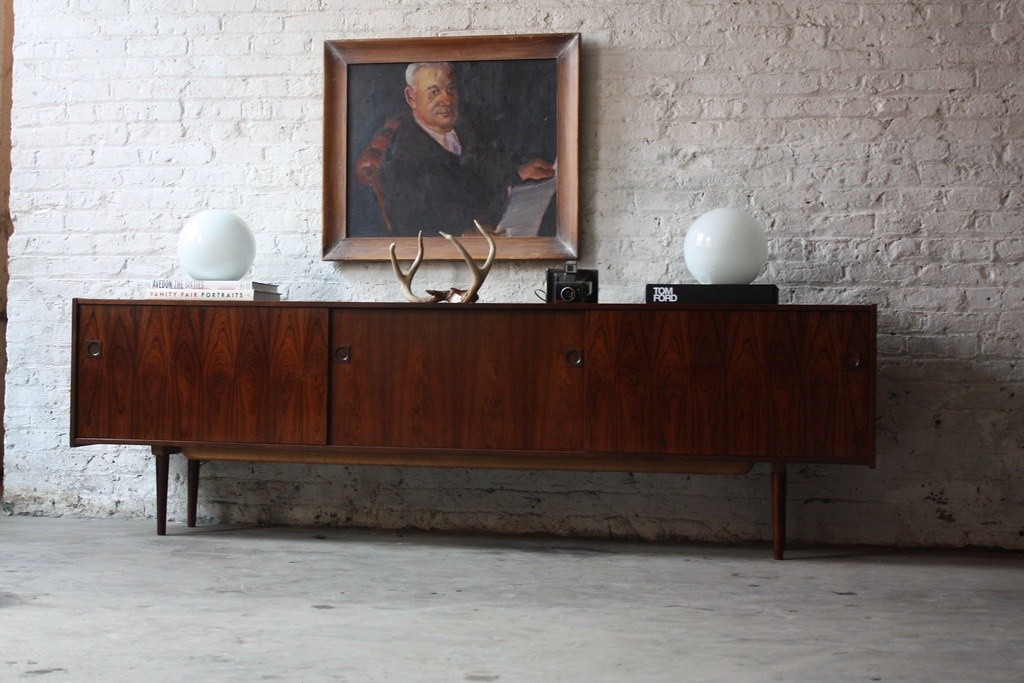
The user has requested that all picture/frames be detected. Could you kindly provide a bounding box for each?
[321,33,579,261]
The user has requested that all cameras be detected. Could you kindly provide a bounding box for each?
[545,261,599,302]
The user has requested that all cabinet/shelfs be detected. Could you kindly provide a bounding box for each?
[70,298,878,561]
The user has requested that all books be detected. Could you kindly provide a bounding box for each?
[148,279,283,300]
